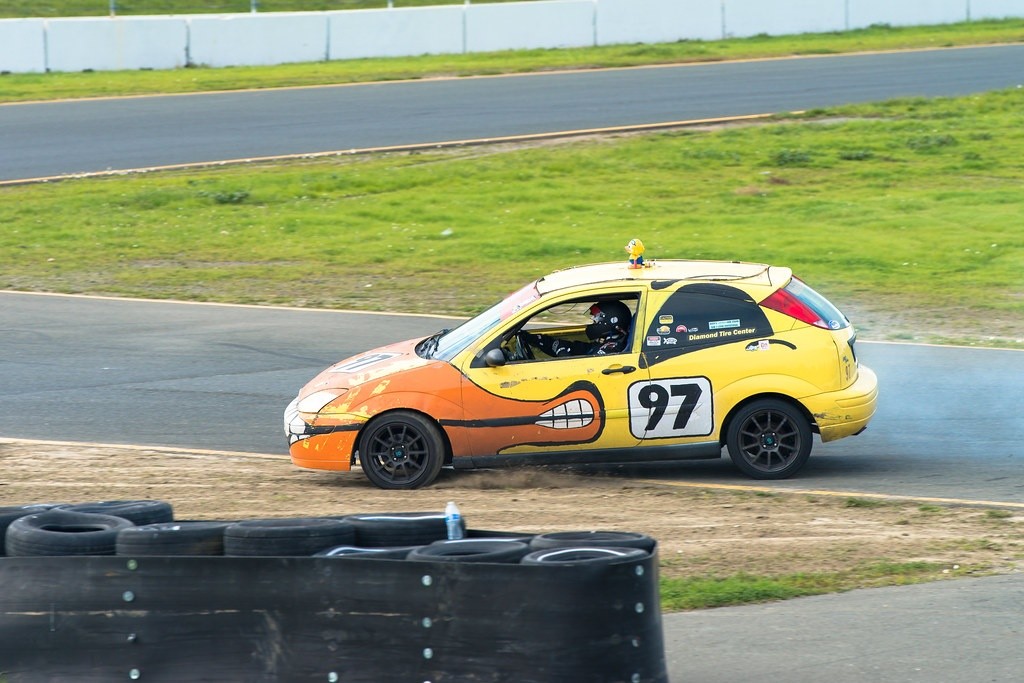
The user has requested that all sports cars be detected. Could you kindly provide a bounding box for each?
[282,237,880,491]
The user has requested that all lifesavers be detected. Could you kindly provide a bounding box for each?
[0,497,658,569]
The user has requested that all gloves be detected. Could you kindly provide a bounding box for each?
[515,330,537,346]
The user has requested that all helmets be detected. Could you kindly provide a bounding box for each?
[585,299,632,340]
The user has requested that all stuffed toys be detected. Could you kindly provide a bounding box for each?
[625,238,644,269]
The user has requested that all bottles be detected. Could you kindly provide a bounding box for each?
[444,501,463,540]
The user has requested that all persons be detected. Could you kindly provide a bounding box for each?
[519,301,633,357]
